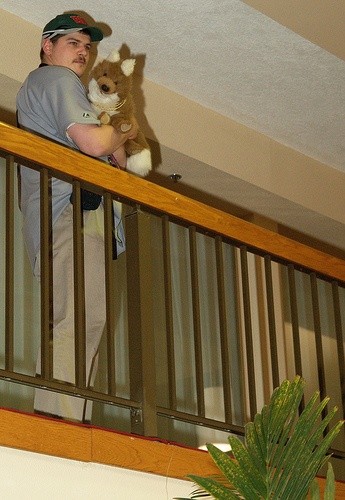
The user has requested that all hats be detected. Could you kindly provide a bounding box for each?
[42,14,103,41]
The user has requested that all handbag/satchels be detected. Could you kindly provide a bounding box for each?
[70,187,101,210]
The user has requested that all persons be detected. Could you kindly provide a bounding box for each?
[16,10,139,424]
